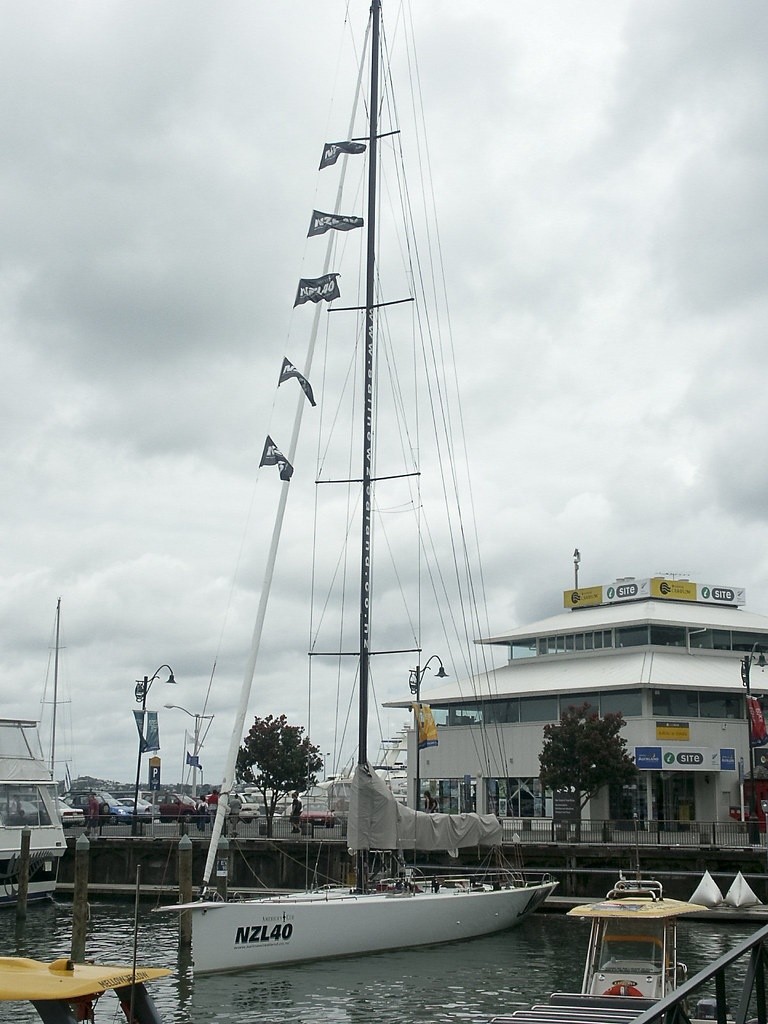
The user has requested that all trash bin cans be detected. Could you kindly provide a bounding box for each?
[556,827,567,841]
[342,820,347,836]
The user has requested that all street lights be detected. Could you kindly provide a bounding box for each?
[131,664,175,843]
[163,704,215,802]
[742,642,768,845]
[405,655,451,811]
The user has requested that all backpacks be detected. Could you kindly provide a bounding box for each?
[200,803,206,812]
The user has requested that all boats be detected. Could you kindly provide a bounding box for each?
[565,878,707,996]
[0,718,70,905]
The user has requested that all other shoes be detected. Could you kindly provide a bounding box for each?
[291,829,300,833]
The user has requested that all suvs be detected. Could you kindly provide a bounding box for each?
[57,790,134,824]
[230,792,260,823]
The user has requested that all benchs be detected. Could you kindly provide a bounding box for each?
[487,992,664,1024]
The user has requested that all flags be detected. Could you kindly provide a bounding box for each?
[292,273,341,309]
[186,750,198,766]
[186,732,204,748]
[278,357,317,407]
[319,141,366,169]
[306,210,364,238]
[259,435,294,481]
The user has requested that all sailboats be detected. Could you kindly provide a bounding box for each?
[152,0,564,978]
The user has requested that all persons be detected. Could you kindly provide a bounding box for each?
[290,792,303,833]
[198,791,242,835]
[424,791,437,813]
[86,794,97,837]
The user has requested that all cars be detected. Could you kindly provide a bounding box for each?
[137,792,209,822]
[117,797,153,817]
[300,798,334,828]
[24,800,85,827]
